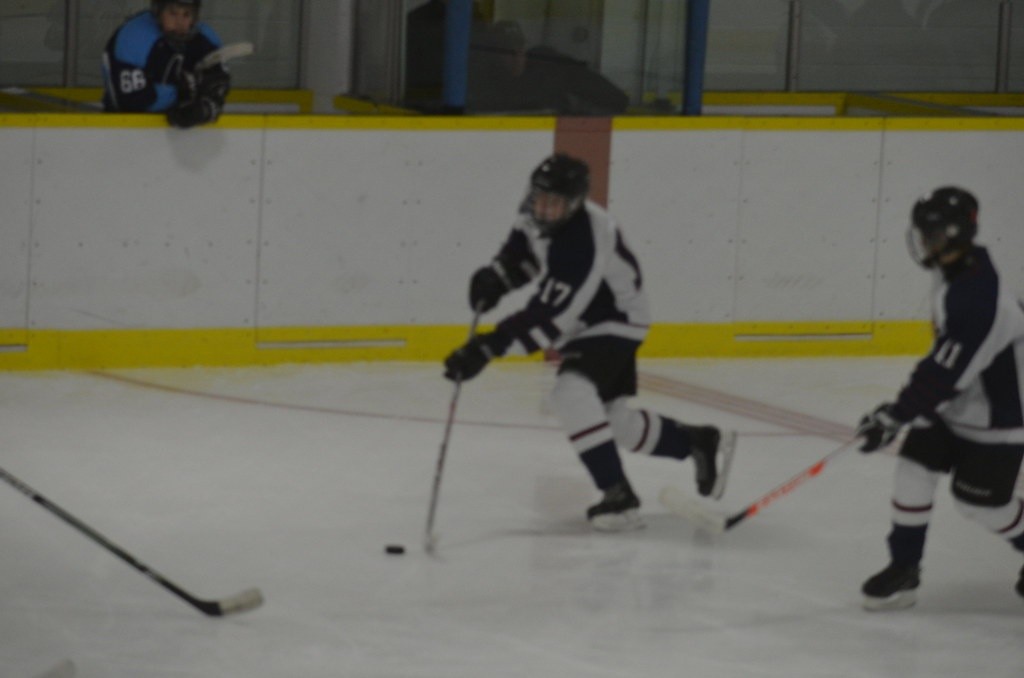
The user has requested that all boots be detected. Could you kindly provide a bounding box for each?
[628,410,738,500]
[995,498,1024,598]
[859,499,933,610]
[571,422,643,531]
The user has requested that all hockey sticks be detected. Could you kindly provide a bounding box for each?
[192,39,256,70]
[421,303,487,554]
[722,436,862,533]
[1,464,266,621]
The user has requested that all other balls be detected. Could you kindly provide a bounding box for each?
[385,545,404,554]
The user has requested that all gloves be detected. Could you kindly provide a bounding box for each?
[469,267,507,313]
[442,332,490,382]
[166,96,212,127]
[858,403,902,452]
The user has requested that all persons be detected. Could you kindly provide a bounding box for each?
[443,153,736,531]
[406,0,628,117]
[101,0,228,128]
[855,188,1024,610]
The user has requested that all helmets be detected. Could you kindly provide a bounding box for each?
[907,187,979,270]
[530,154,590,229]
[153,0,201,42]
